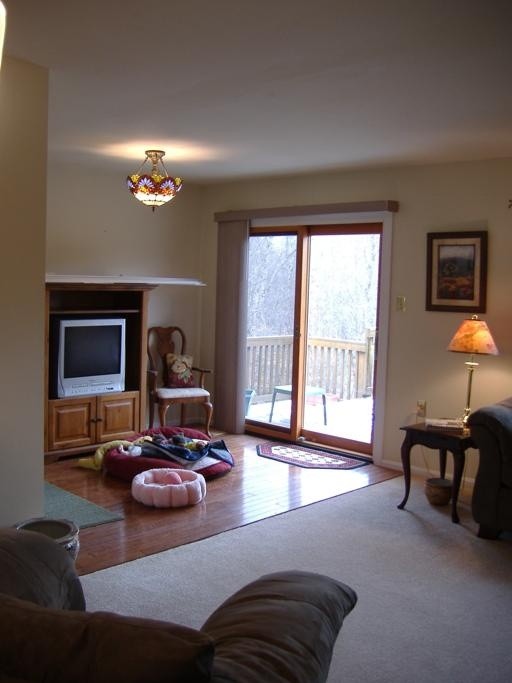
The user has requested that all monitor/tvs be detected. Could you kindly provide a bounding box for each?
[50,317,127,399]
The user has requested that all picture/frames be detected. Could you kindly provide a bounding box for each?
[422,230,487,315]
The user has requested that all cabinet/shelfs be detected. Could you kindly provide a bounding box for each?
[44,277,150,466]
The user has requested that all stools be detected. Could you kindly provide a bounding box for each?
[266,383,328,428]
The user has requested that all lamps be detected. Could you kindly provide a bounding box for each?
[441,316,501,429]
[123,145,186,214]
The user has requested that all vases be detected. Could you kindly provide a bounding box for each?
[424,477,453,510]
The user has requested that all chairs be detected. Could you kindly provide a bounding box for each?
[144,324,216,442]
[0,522,363,682]
[468,393,511,540]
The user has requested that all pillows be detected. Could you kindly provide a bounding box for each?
[163,351,197,388]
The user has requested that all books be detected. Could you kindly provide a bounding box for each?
[424,417,464,433]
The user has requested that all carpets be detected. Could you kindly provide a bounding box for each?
[78,470,512,683]
[256,439,372,471]
[43,476,126,533]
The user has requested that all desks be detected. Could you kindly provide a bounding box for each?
[396,421,477,528]
